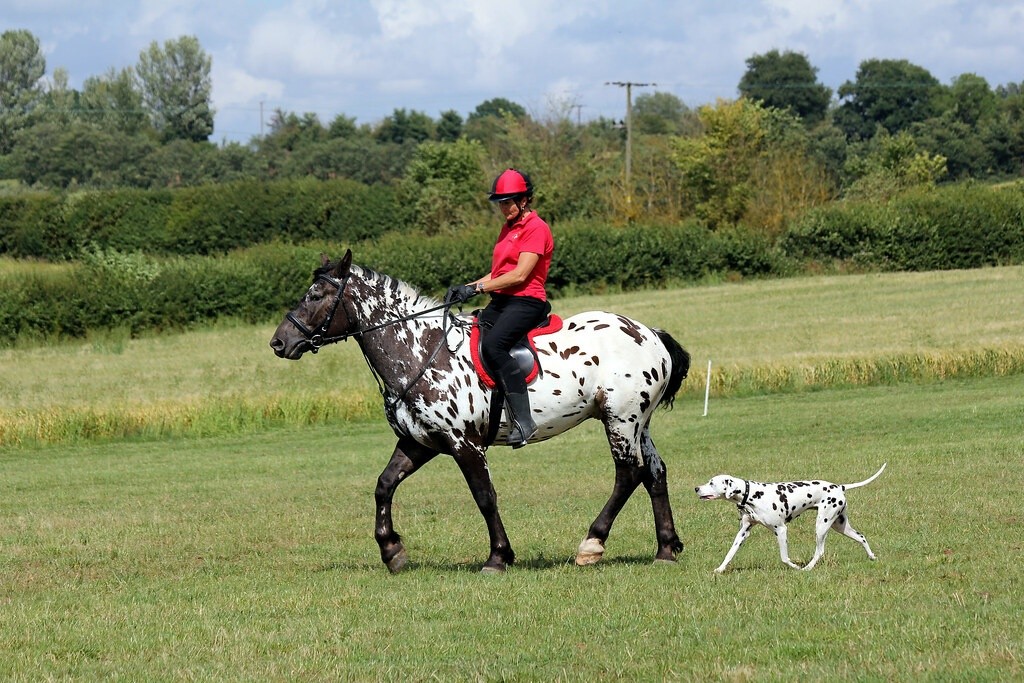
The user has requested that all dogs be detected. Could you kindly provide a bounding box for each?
[694,462,889,574]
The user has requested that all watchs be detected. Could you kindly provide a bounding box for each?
[478,282,485,294]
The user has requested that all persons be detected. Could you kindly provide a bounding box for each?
[442,166,554,447]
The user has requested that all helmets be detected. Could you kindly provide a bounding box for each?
[488,168,533,201]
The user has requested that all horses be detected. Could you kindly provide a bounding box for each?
[269,247,692,575]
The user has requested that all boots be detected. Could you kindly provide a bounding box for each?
[504,389,538,445]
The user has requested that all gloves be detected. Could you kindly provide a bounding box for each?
[445,283,478,303]
